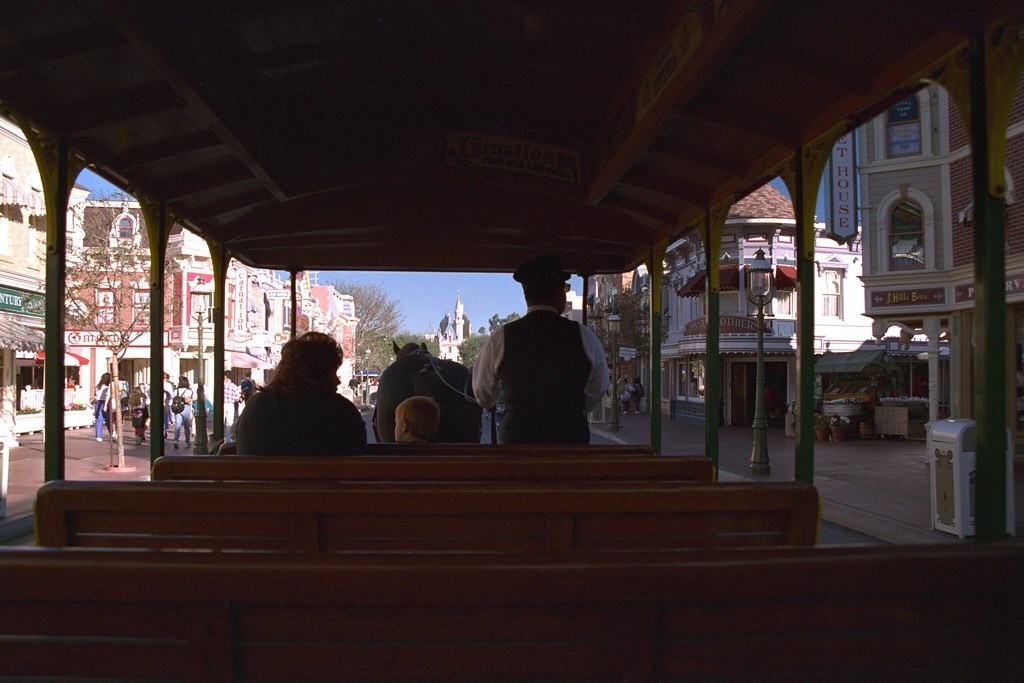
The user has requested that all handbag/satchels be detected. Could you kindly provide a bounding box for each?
[121,389,130,408]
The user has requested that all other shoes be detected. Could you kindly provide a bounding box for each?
[135,438,142,447]
[112,431,116,437]
[141,435,147,442]
[96,437,102,442]
[111,433,119,440]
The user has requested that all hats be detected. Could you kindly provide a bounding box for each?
[512,253,571,283]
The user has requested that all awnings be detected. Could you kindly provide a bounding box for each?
[225,351,274,370]
[0,317,70,353]
[677,266,796,298]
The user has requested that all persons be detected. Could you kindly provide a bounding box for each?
[394,396,441,443]
[224,370,239,441]
[230,331,367,456]
[172,376,197,449]
[349,376,358,397]
[103,371,130,437]
[192,398,213,412]
[191,380,198,437]
[94,374,117,441]
[471,258,610,445]
[163,372,175,429]
[127,383,150,445]
[765,383,776,419]
[234,370,257,419]
[621,378,644,414]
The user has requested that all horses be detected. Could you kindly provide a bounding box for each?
[373,341,483,443]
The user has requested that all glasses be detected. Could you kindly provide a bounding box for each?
[556,282,572,293]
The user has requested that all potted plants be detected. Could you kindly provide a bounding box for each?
[814,417,830,442]
[859,411,876,438]
[830,415,850,442]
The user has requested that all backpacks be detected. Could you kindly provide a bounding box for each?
[172,388,186,413]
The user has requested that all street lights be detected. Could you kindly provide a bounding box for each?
[608,314,622,433]
[745,246,774,479]
[364,349,372,410]
[187,273,214,456]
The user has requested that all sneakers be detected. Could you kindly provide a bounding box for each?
[184,442,191,448]
[635,411,641,414]
[623,410,628,415]
[173,439,179,449]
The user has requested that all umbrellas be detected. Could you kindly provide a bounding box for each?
[15,349,89,366]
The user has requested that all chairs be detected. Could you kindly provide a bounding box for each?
[0,442,1024,683]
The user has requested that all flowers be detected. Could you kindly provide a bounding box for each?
[17,403,87,415]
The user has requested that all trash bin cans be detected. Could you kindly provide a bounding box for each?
[341,389,353,403]
[929,418,1015,540]
[0,418,10,518]
